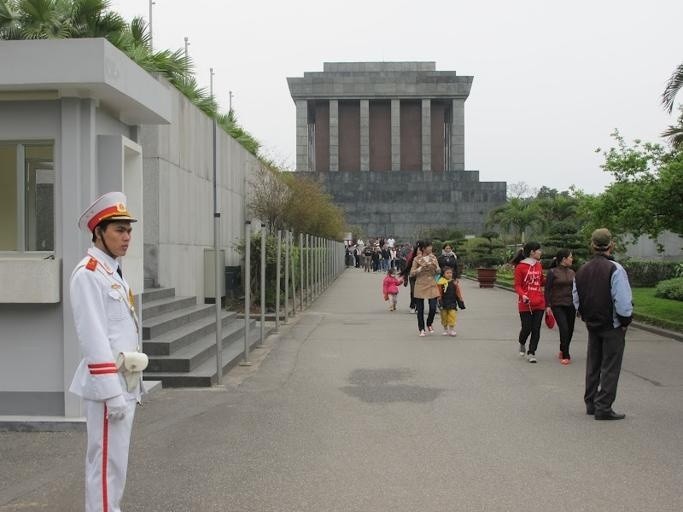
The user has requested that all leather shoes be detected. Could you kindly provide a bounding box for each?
[595,410,625,421]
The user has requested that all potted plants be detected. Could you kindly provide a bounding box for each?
[468,229,509,289]
[431,227,467,278]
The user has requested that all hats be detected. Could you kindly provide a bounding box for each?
[591,228,612,249]
[78,191,138,234]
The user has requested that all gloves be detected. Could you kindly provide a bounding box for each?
[105,395,127,421]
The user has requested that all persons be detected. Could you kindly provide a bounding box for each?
[570,227,633,420]
[67,190,150,511]
[343,235,467,339]
[542,249,581,367]
[508,240,547,364]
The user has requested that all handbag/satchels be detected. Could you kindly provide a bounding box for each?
[545,311,554,328]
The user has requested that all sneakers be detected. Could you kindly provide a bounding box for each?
[527,353,536,362]
[419,330,424,336]
[449,330,456,336]
[519,343,525,356]
[442,329,448,335]
[427,326,432,332]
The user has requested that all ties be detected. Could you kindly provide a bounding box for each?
[117,265,122,279]
